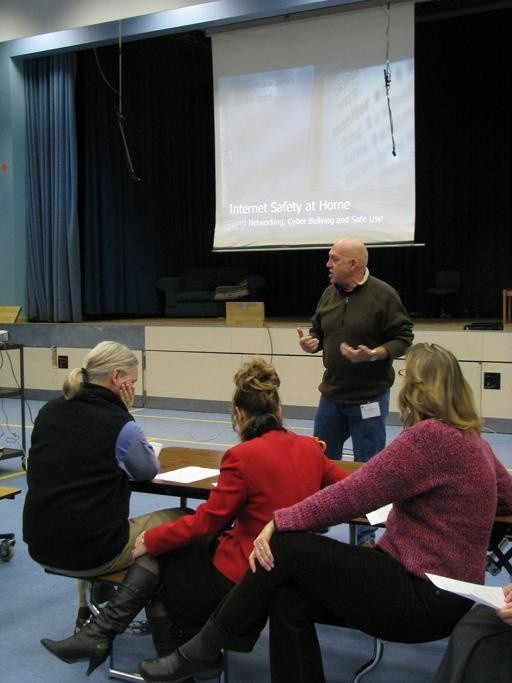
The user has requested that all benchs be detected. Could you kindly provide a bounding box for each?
[44,563,383,681]
[2,486,23,564]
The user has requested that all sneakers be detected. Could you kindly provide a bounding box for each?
[74,616,89,637]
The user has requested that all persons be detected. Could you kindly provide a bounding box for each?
[19,338,196,634]
[296,236,414,550]
[138,339,511,683]
[430,572,512,683]
[40,353,356,681]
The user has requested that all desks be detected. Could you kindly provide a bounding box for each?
[129,446,510,577]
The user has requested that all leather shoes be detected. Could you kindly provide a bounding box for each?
[138,649,225,682]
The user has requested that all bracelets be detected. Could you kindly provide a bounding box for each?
[370,349,378,362]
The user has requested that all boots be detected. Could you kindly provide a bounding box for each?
[39,560,160,677]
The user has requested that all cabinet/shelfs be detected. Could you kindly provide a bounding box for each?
[1,330,28,472]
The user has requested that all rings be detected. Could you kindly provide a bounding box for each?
[257,546,267,551]
